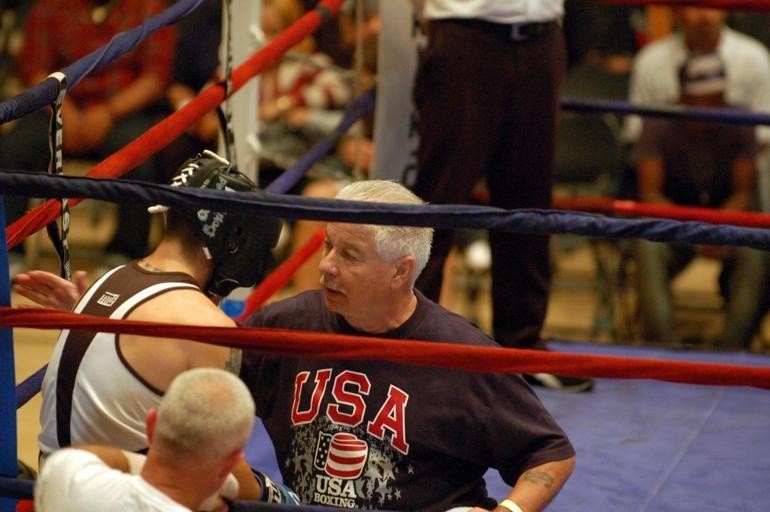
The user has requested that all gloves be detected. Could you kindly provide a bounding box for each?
[249,467,301,506]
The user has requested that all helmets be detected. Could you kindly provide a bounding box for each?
[149,148,285,297]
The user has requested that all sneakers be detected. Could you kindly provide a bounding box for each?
[523,371,594,394]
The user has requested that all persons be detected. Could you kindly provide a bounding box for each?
[1,0,770,512]
